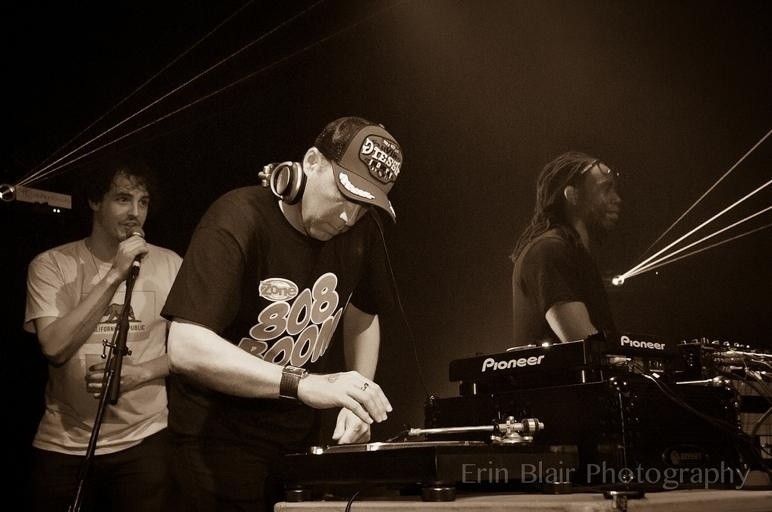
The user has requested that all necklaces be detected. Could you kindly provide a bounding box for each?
[87,244,102,281]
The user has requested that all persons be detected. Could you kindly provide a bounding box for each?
[160,117,403,512]
[21,164,184,512]
[510,151,625,357]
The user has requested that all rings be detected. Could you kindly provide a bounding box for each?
[361,383,368,392]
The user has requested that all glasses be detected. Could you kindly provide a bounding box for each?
[580,158,624,180]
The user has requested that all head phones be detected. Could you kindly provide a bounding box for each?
[270,158,308,204]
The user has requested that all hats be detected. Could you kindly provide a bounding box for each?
[314,116,403,224]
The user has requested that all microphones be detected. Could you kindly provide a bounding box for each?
[126,226,145,273]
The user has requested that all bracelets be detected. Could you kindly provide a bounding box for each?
[278,365,308,405]
